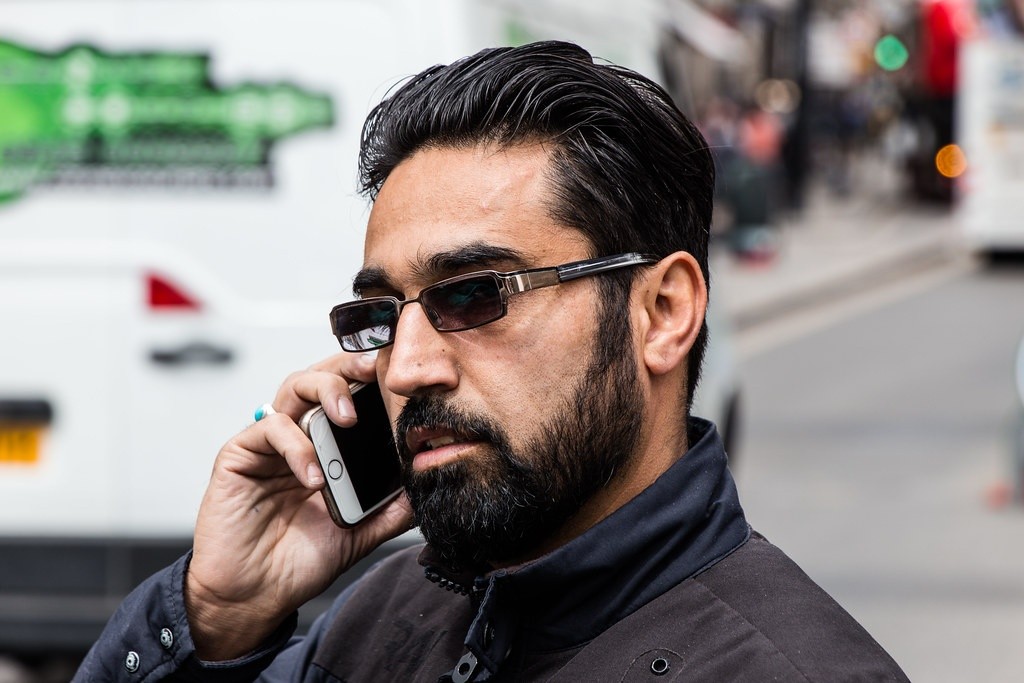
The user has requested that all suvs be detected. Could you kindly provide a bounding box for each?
[0,160,748,657]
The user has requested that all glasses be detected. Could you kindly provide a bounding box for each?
[328,249,666,354]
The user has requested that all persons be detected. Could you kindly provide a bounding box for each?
[69,41,914,683]
[702,83,952,264]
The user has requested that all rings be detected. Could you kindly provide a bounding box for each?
[253,402,277,422]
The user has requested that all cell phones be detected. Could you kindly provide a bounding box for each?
[299,380,406,530]
